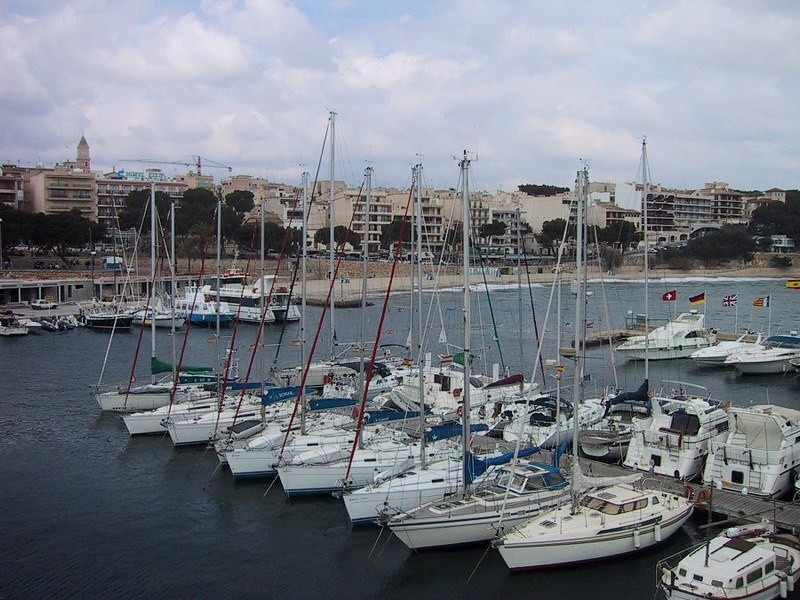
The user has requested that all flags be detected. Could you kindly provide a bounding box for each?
[721,294,737,306]
[402,358,414,366]
[785,280,800,290]
[662,290,676,302]
[688,292,705,306]
[441,355,453,364]
[555,364,564,372]
[752,295,769,307]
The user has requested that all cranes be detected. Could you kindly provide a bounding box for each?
[118,156,231,176]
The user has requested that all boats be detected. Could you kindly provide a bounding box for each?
[171,280,232,324]
[690,331,763,365]
[722,331,800,375]
[656,480,800,600]
[615,310,716,361]
[705,402,800,501]
[303,354,616,451]
[620,379,728,484]
[0,311,84,335]
[211,252,300,324]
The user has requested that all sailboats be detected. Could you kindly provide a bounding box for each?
[270,164,467,498]
[87,188,134,328]
[579,137,660,462]
[339,161,523,522]
[377,150,611,549]
[94,107,371,478]
[126,206,186,330]
[489,165,696,570]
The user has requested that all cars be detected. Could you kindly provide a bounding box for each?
[31,300,58,310]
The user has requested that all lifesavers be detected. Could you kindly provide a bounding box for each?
[454,388,460,397]
[697,490,711,510]
[457,406,463,417]
[685,486,694,499]
